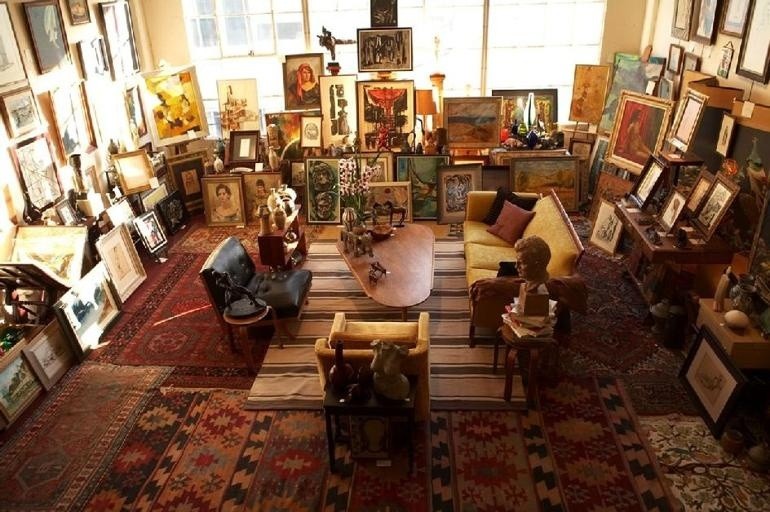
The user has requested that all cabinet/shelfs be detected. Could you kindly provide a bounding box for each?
[615,200,734,326]
[692,295,769,370]
[256,204,308,269]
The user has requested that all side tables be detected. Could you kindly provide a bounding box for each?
[493,327,553,402]
[222,302,283,374]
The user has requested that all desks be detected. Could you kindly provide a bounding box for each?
[321,377,417,473]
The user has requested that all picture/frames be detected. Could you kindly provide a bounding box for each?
[65,2,93,26]
[136,211,168,255]
[289,159,305,188]
[243,172,284,227]
[48,83,94,161]
[74,165,103,194]
[370,0,399,28]
[304,155,342,226]
[201,176,245,227]
[51,260,124,366]
[98,2,139,81]
[338,150,394,224]
[718,1,752,38]
[568,64,610,126]
[693,0,722,46]
[491,89,559,147]
[0,338,43,423]
[665,87,710,156]
[139,65,210,149]
[75,36,108,82]
[142,183,170,234]
[603,88,674,178]
[21,318,77,391]
[666,44,681,75]
[57,201,78,227]
[627,155,669,214]
[681,169,716,219]
[319,74,358,154]
[168,156,211,215]
[396,155,449,220]
[441,96,504,149]
[681,52,701,82]
[0,86,43,141]
[677,324,750,443]
[283,53,326,112]
[735,1,770,85]
[106,197,138,237]
[110,149,156,197]
[496,151,568,167]
[229,130,260,164]
[689,170,741,242]
[585,169,634,226]
[362,181,414,222]
[438,162,485,226]
[95,222,147,305]
[509,155,581,214]
[125,83,152,149]
[23,1,73,75]
[715,110,737,160]
[671,0,695,42]
[300,115,324,149]
[355,79,415,154]
[657,186,687,238]
[217,79,260,143]
[7,132,65,214]
[587,198,623,257]
[356,27,414,72]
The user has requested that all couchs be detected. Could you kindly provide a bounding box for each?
[313,310,432,417]
[462,191,585,348]
[199,237,312,350]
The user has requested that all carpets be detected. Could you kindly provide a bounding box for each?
[246,240,529,410]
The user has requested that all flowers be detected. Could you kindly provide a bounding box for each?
[340,153,383,207]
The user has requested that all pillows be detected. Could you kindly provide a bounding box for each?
[485,199,535,247]
[479,186,536,226]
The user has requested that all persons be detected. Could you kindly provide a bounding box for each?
[256,179,270,208]
[514,235,551,316]
[213,184,240,221]
[628,110,651,160]
[288,63,319,107]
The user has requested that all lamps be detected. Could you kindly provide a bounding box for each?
[416,89,434,152]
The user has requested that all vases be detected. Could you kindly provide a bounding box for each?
[342,206,357,226]
